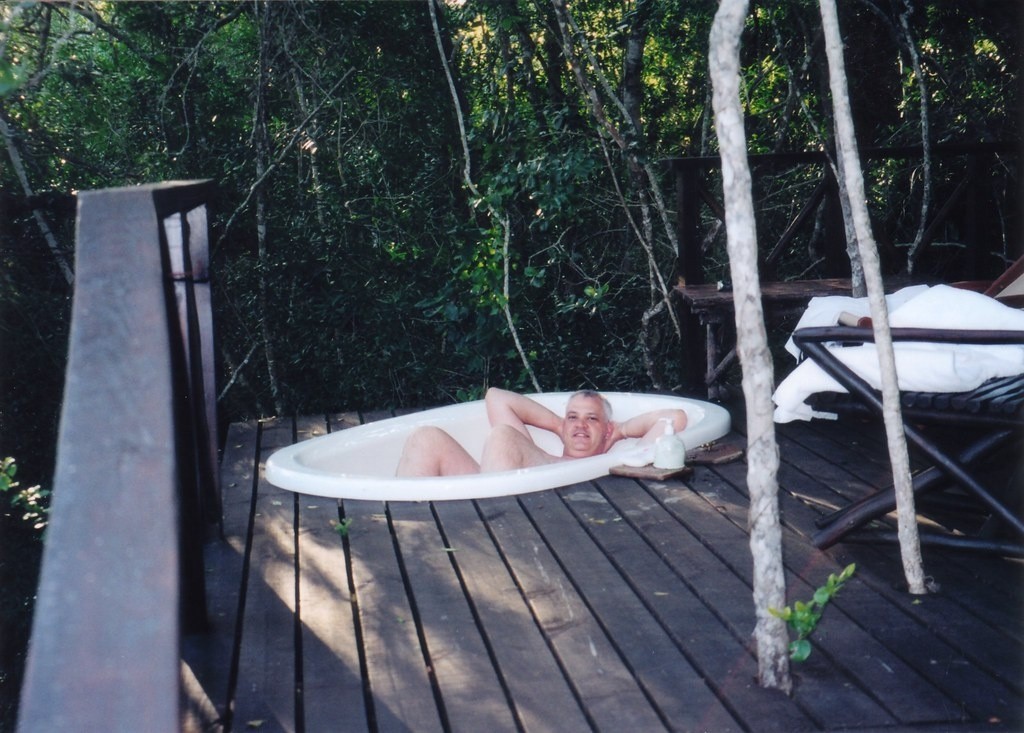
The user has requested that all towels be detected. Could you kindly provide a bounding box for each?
[769,283,1023,426]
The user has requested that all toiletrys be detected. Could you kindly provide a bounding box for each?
[653,418,686,469]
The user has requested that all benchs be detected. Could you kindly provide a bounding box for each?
[660,143,1006,407]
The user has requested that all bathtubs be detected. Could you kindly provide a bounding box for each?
[263,388,730,500]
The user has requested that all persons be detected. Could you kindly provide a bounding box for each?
[396,388,688,478]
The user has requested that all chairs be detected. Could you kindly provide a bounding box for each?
[791,291,1024,557]
